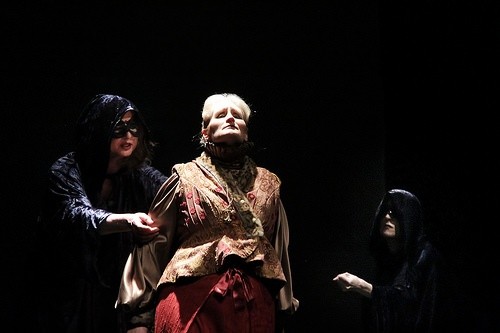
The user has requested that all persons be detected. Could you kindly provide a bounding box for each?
[333,189,459,333]
[47,94,169,333]
[114,93,299,333]
